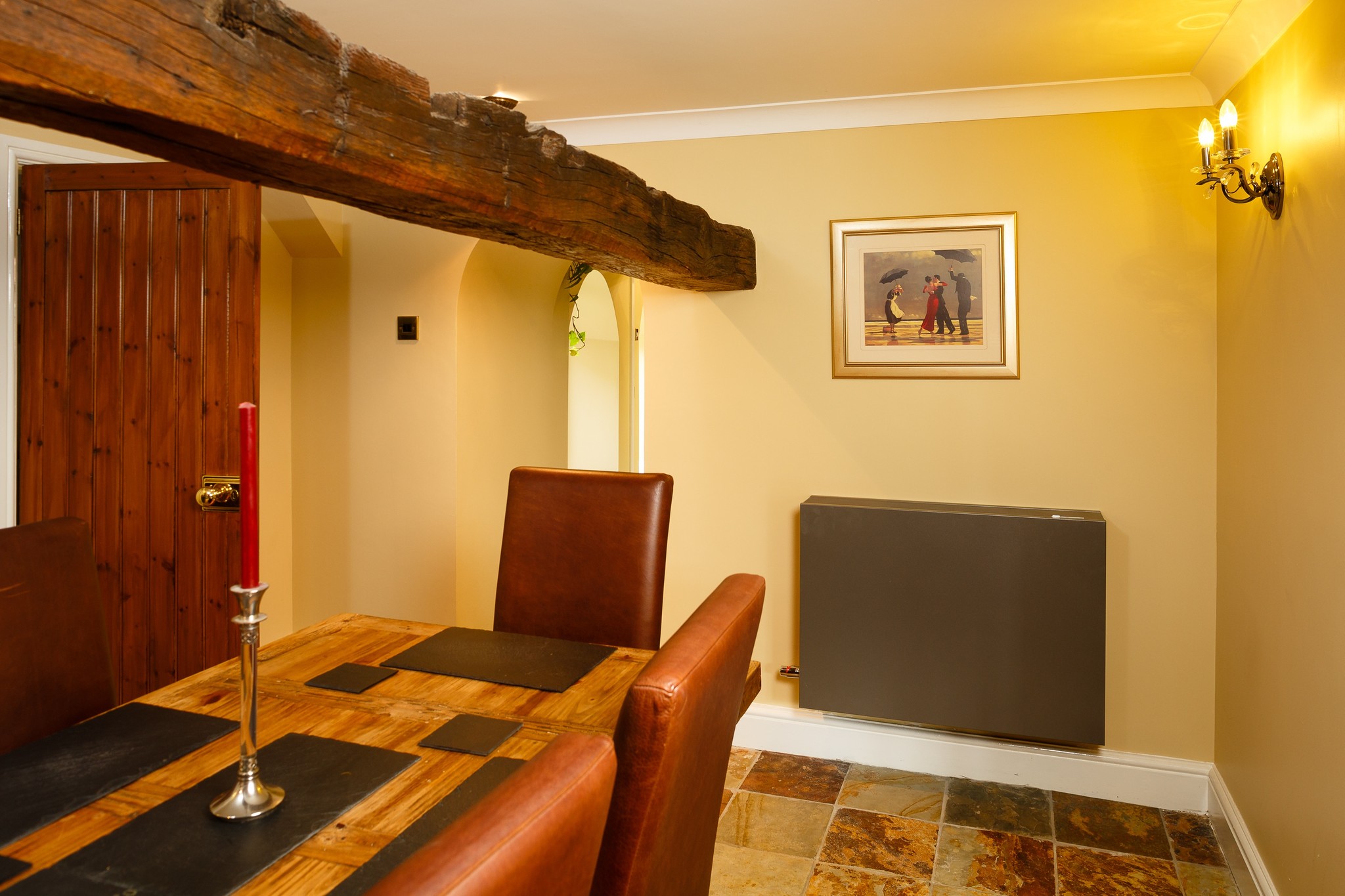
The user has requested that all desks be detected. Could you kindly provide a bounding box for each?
[1,610,657,895]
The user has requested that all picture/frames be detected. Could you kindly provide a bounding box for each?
[829,210,1021,380]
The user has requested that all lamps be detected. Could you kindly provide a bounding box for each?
[1192,98,1284,220]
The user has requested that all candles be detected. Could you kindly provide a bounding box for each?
[237,403,264,589]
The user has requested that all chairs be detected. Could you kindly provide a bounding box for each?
[1,514,119,754]
[490,464,674,651]
[592,574,768,895]
